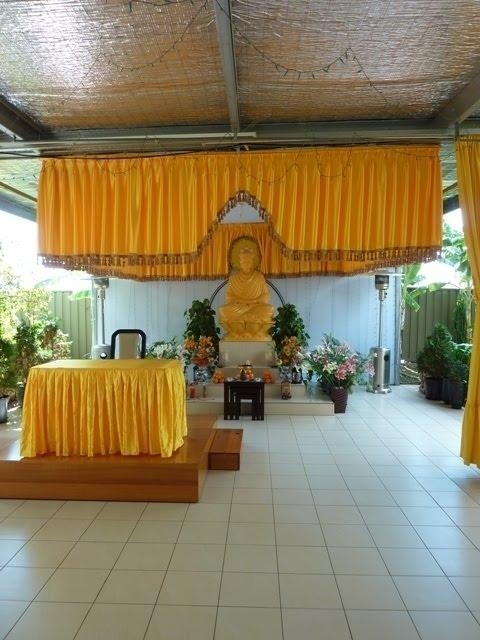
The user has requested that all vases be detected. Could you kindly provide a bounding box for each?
[331,387,347,413]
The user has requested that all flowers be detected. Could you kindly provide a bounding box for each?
[302,334,376,387]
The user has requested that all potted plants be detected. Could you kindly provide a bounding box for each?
[417,323,473,409]
[0,338,20,423]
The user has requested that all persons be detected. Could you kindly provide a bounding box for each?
[218,248,274,322]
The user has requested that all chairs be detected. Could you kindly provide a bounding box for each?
[110,329,146,358]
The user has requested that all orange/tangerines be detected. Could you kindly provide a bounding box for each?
[212,370,224,384]
[263,372,273,383]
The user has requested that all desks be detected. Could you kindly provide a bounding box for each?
[21,359,188,455]
[223,378,264,420]
